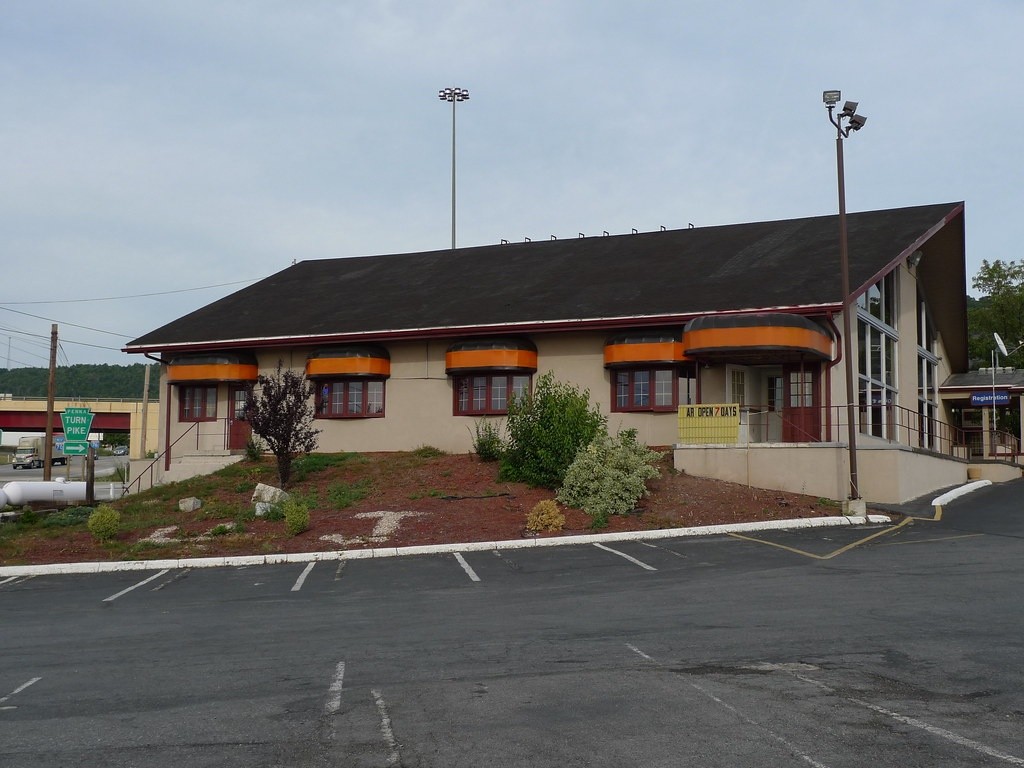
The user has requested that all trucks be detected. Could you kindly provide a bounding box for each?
[12,434,73,469]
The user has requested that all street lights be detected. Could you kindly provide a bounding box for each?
[439,86,470,250]
[821,88,869,522]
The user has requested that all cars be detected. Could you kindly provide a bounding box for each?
[113,446,129,457]
[86,449,99,461]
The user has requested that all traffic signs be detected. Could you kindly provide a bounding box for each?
[60,408,95,456]
